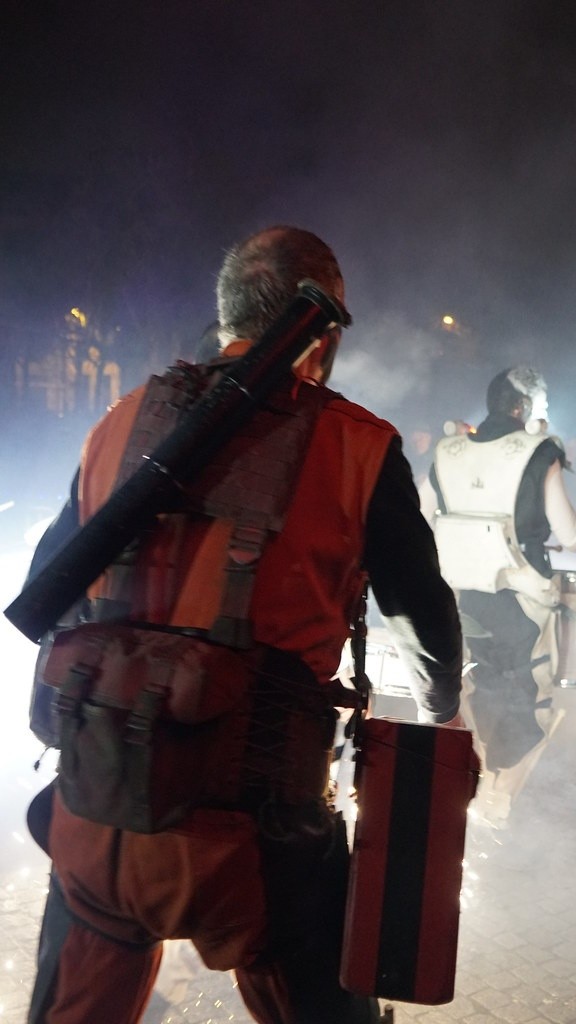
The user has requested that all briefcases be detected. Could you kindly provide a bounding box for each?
[339,714,478,1009]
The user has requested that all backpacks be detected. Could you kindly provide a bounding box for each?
[51,618,236,831]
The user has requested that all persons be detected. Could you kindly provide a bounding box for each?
[6,226,483,1024]
[416,361,576,846]
